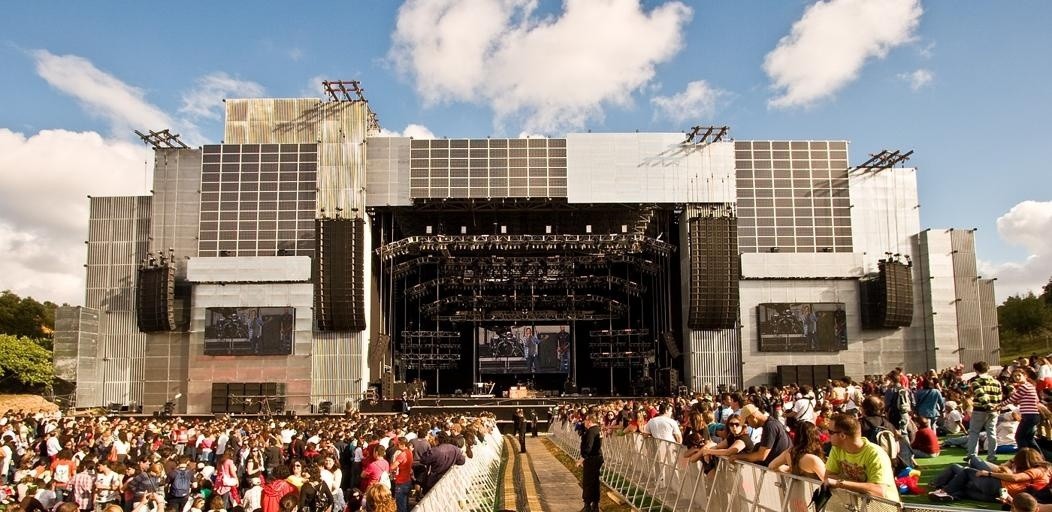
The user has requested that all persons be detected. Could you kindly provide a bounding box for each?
[246,310,265,353]
[801,306,821,352]
[833,304,846,346]
[281,308,292,352]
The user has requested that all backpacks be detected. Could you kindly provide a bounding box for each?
[173,468,190,497]
[866,417,899,470]
[374,462,392,490]
[53,461,70,483]
[895,384,913,414]
[309,479,329,512]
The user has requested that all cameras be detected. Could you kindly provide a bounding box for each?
[999,488,1008,499]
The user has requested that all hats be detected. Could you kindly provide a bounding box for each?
[887,369,900,382]
[740,403,759,425]
[915,414,929,425]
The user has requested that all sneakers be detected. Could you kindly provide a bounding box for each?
[928,489,954,504]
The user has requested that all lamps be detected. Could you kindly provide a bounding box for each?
[691,204,733,219]
[319,205,361,221]
[138,247,177,269]
[375,234,678,261]
[878,249,913,267]
[366,206,375,215]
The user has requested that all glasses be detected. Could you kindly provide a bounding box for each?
[828,428,841,433]
[728,423,740,427]
[252,449,257,452]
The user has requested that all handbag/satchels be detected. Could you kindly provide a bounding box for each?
[222,476,238,486]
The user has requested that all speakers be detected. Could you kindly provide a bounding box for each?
[812,365,829,387]
[245,383,261,413]
[878,263,913,328]
[211,383,227,413]
[688,216,738,330]
[797,365,812,388]
[829,365,845,383]
[777,365,797,387]
[261,382,277,413]
[314,218,366,331]
[228,383,244,414]
[137,267,176,332]
